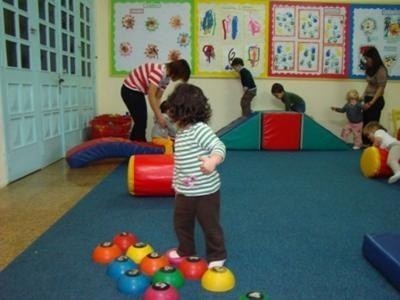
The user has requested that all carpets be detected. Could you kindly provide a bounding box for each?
[0,142,399,300]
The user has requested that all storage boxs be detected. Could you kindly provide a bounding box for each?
[89,113,134,140]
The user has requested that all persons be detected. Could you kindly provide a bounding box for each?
[231,57,256,116]
[121,59,191,141]
[271,83,305,113]
[330,90,369,150]
[166,83,227,271]
[359,45,388,149]
[362,121,400,185]
[151,101,176,157]
